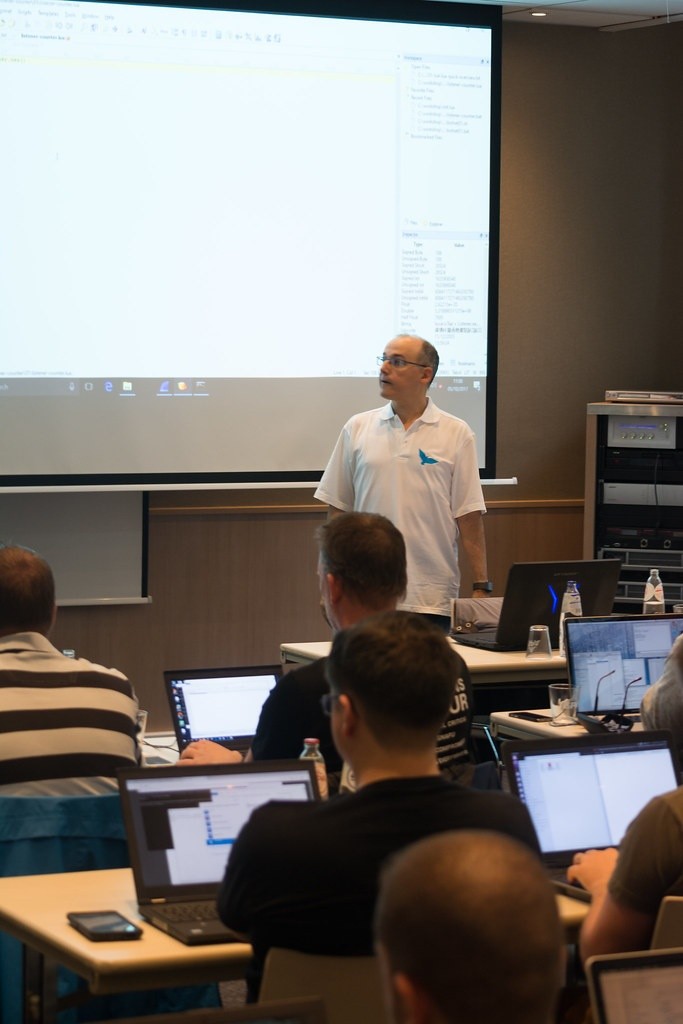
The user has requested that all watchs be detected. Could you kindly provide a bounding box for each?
[472,581,494,593]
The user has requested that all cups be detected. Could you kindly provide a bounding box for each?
[549,683,580,724]
[673,604,683,613]
[136,709,147,749]
[526,625,552,661]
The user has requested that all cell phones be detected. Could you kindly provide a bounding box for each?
[509,712,551,722]
[66,910,142,942]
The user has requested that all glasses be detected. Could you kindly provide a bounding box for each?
[591,670,642,733]
[376,356,426,368]
[322,692,358,717]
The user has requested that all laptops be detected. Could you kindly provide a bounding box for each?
[451,559,622,652]
[500,730,683,904]
[584,945,683,1024]
[115,759,323,946]
[164,664,283,762]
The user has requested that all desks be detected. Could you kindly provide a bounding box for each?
[280,631,571,706]
[490,708,647,752]
[0,866,593,1023]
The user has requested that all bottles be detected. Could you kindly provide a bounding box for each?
[300,738,328,800]
[63,649,75,659]
[643,569,665,614]
[560,581,582,656]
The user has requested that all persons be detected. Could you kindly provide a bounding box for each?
[311,337,488,638]
[0,542,149,798]
[375,828,571,1024]
[215,611,542,1003]
[177,512,476,774]
[552,633,683,976]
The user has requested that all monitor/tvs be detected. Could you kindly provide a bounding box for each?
[562,613,683,716]
